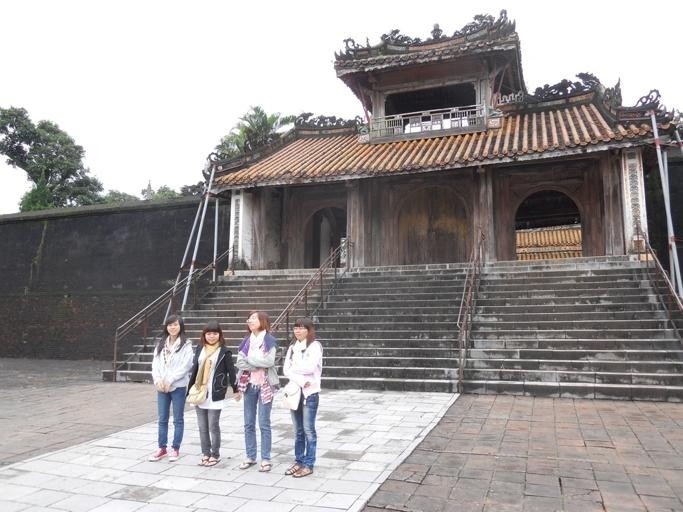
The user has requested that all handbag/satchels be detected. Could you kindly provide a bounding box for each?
[275,386,301,410]
[185,382,207,403]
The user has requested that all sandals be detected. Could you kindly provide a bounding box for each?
[285,463,302,474]
[293,468,312,477]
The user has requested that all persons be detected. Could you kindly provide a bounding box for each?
[234,311,281,472]
[150,315,194,461]
[185,321,242,467]
[282,316,324,478]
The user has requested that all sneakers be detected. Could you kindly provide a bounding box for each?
[149,447,168,461]
[169,447,179,460]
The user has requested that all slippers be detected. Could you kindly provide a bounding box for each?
[240,460,257,469]
[259,462,273,472]
[199,455,221,466]
